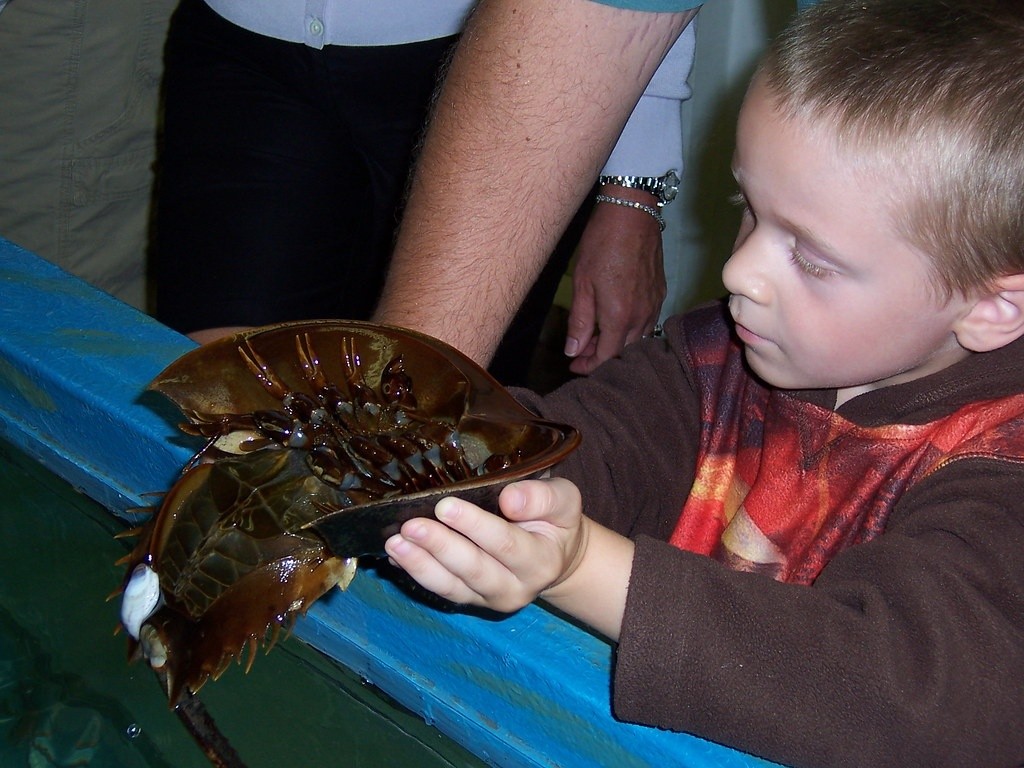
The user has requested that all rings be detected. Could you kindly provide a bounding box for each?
[642,336,654,340]
[654,324,663,336]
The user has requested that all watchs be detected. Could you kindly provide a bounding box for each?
[596,170,682,207]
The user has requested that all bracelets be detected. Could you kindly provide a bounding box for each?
[596,195,666,232]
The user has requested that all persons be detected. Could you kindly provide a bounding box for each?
[187,0,708,371]
[384,0,1024,768]
[0,0,182,313]
[146,0,696,374]
